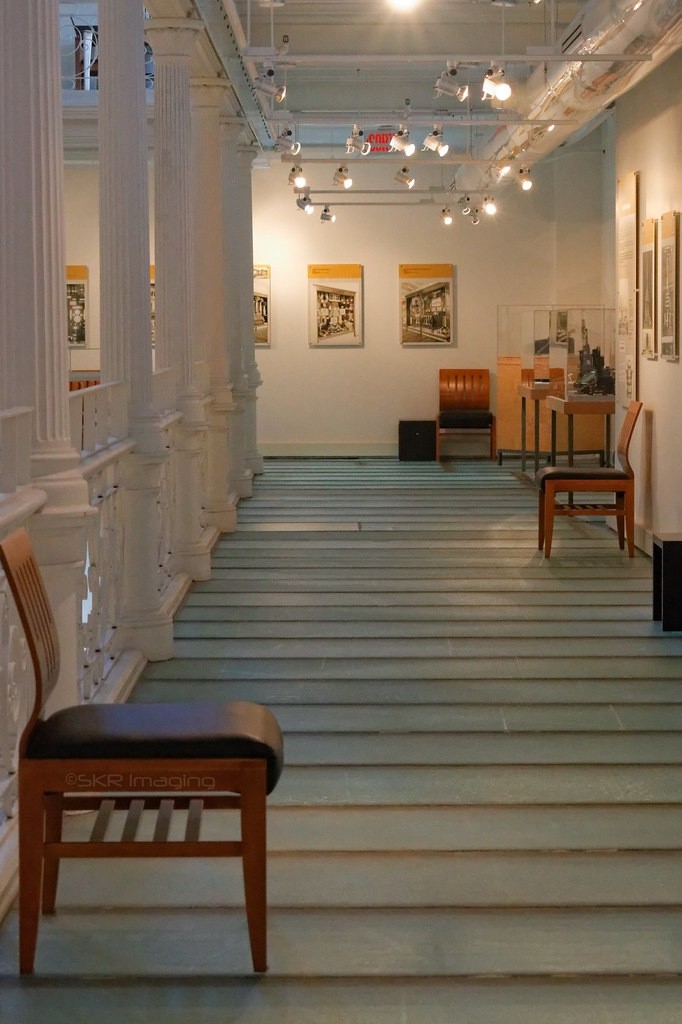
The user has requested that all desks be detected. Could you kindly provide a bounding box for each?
[496,354,611,468]
[516,384,573,476]
[546,396,616,519]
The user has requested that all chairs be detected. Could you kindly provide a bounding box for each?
[436,369,497,464]
[537,400,643,560]
[0,527,285,980]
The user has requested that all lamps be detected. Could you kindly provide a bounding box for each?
[254,62,534,224]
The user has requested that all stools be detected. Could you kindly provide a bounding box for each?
[651,533,682,631]
